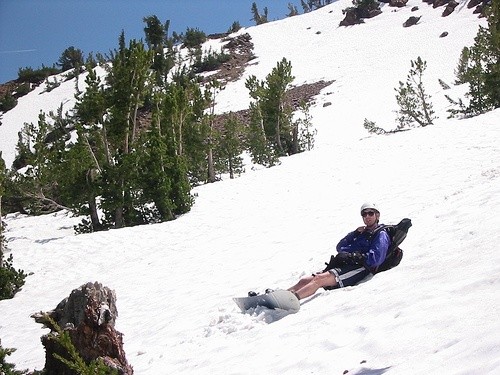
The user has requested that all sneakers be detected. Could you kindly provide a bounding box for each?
[248,291,258,296]
[266,288,274,293]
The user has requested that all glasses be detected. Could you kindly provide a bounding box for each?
[362,211,376,216]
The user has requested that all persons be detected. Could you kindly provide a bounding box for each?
[248,201,390,301]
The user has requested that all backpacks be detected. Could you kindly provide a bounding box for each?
[359,218,412,274]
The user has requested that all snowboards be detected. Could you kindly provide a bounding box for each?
[231,289,300,314]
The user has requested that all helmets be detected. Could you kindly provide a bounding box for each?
[361,201,380,213]
